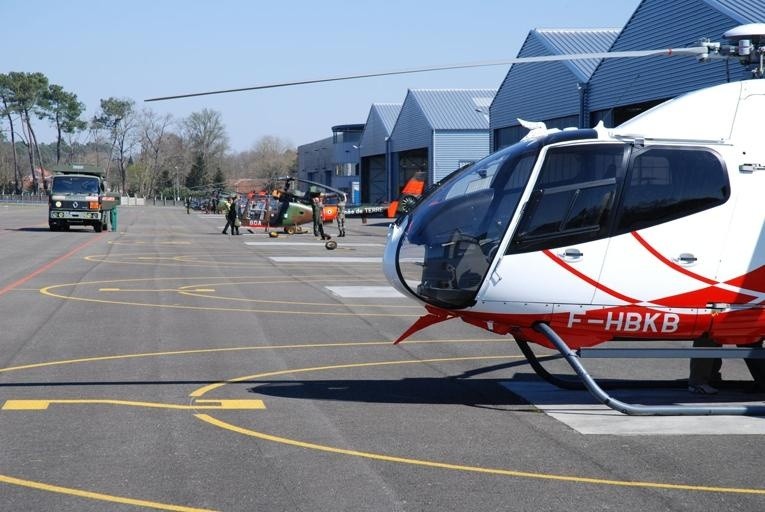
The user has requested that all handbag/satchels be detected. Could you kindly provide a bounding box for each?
[234,217,241,227]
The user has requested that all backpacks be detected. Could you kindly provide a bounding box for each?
[216,199,227,211]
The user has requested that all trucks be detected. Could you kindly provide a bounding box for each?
[48,162,122,232]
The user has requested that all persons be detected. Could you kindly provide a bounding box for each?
[335,193,347,238]
[312,196,325,236]
[221,195,243,236]
[107,203,117,235]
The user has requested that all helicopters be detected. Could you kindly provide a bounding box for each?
[223,166,431,238]
[143,20,765,417]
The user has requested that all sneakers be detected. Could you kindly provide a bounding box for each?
[221,230,240,235]
[314,231,320,236]
[320,235,331,240]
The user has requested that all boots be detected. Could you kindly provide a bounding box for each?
[337,230,346,237]
